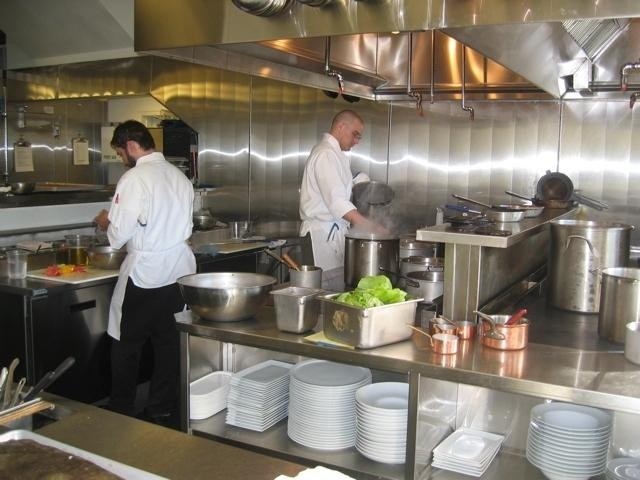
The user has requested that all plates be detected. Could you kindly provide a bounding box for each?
[189,357,410,466]
[430,426,505,478]
[524,402,640,480]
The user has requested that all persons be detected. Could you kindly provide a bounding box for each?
[297,109,390,272]
[94,120,197,415]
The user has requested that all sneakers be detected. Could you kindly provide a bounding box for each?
[144,405,178,428]
[99,405,132,416]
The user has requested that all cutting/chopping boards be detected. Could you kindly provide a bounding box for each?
[25,264,122,286]
[194,239,275,254]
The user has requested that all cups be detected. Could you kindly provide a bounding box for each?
[5,250,30,280]
[624,320,640,366]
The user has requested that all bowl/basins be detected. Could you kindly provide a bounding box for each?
[82,246,129,269]
[193,215,218,229]
[7,181,36,196]
[175,271,278,324]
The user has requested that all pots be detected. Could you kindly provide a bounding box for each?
[342,230,444,305]
[428,317,457,335]
[549,219,636,314]
[404,323,459,355]
[470,309,533,350]
[592,267,640,347]
[439,168,609,225]
[265,249,324,289]
[436,314,475,340]
